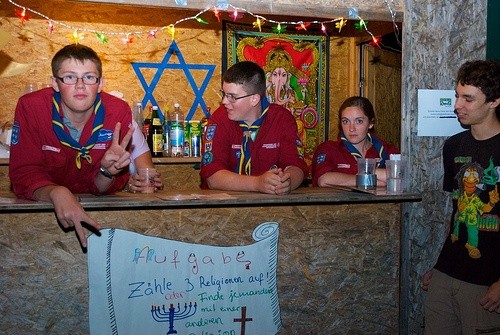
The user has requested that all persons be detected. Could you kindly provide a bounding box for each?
[421,59,500,335]
[311,95,399,187]
[8,43,135,248]
[125,120,162,193]
[198,61,309,194]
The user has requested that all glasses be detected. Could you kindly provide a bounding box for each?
[53,74,101,85]
[219,88,257,102]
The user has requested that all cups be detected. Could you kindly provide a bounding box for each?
[385,160,407,192]
[358,158,376,190]
[24,84,38,94]
[138,169,156,193]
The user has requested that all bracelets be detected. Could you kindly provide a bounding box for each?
[99,168,114,179]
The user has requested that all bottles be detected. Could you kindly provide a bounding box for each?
[170,103,184,158]
[143,106,152,149]
[162,107,172,157]
[135,102,144,134]
[151,106,164,158]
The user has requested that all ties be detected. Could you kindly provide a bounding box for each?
[53,91,106,170]
[235,95,269,176]
[341,131,386,172]
